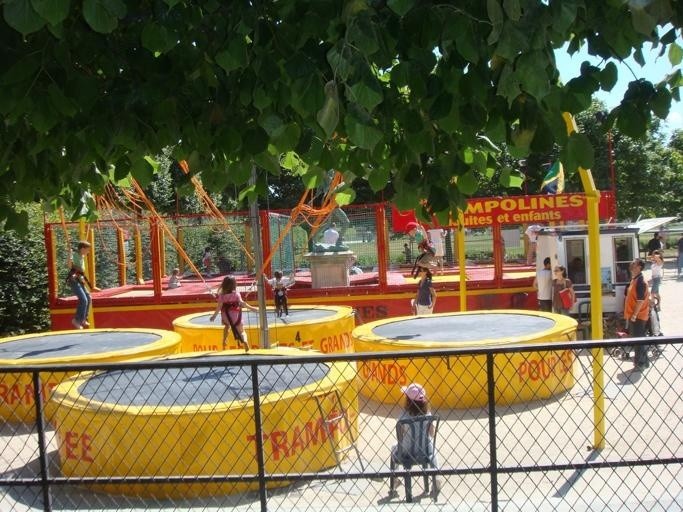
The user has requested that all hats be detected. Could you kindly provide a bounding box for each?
[400,383,426,402]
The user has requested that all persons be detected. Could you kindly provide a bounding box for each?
[647,249,664,310]
[622,257,651,374]
[324,222,340,244]
[401,242,413,265]
[646,231,683,281]
[168,267,184,288]
[402,221,436,280]
[261,270,296,320]
[208,275,257,353]
[532,256,552,312]
[615,239,630,278]
[410,266,437,315]
[387,381,441,492]
[569,257,584,283]
[549,264,575,317]
[202,246,212,278]
[66,240,101,330]
[349,261,363,275]
[425,227,448,276]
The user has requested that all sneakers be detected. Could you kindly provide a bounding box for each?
[629,360,650,373]
[395,478,402,490]
[431,477,442,490]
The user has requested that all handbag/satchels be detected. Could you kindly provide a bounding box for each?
[558,287,578,310]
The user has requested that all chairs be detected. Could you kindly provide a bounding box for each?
[390,416,440,504]
[576,301,607,340]
